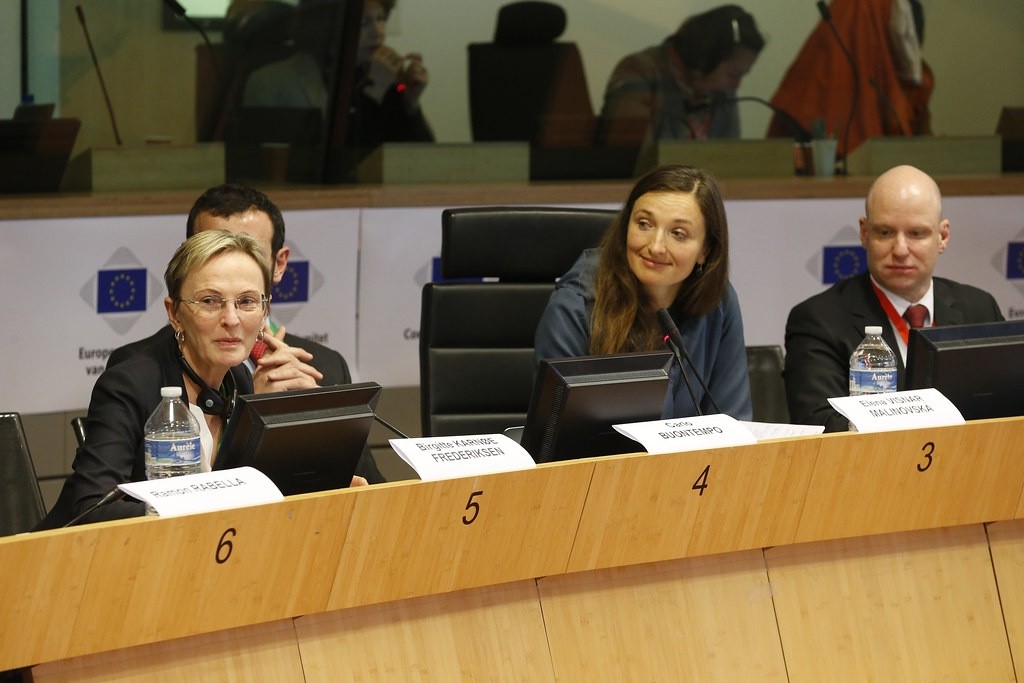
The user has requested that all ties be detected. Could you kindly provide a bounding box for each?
[901,304,928,330]
[249,338,268,369]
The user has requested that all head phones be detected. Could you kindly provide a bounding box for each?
[178,349,238,419]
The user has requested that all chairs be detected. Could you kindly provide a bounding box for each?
[419,210,631,450]
[0,412,88,539]
[466,0,599,184]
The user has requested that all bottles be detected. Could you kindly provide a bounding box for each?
[848,325,898,432]
[144,387,202,516]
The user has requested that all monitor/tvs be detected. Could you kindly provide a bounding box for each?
[520,350,675,464]
[211,382,383,496]
[904,320,1024,420]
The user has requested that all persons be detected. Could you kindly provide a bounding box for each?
[536,165,753,423]
[30,229,323,533]
[781,164,1008,433]
[237,0,434,182]
[109,183,383,487]
[590,5,766,152]
[766,0,930,161]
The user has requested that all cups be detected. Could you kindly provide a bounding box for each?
[261,143,289,182]
[811,140,838,176]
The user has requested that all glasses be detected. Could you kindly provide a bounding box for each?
[173,294,269,320]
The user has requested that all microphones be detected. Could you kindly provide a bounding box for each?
[658,308,722,416]
[75,5,124,146]
[684,96,812,177]
[63,487,126,528]
[164,0,224,141]
[817,0,861,150]
